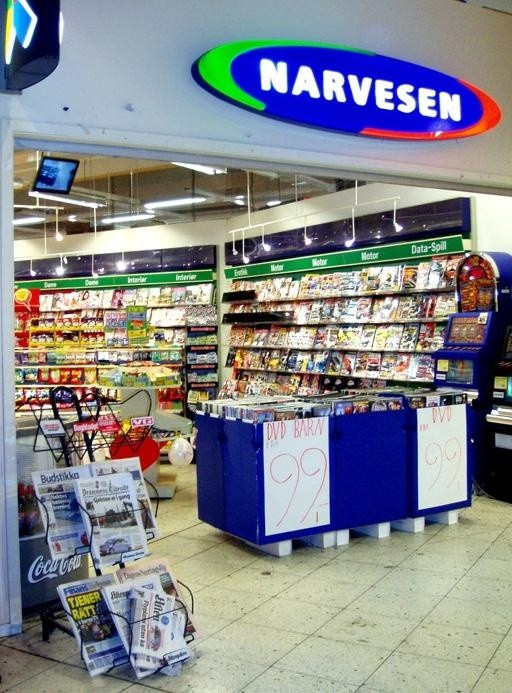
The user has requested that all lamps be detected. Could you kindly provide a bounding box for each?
[345,209,355,248]
[393,200,403,233]
[303,216,312,246]
[242,229,250,263]
[262,226,271,251]
[232,232,238,255]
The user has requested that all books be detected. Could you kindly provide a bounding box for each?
[225,289,467,324]
[194,369,480,424]
[231,347,435,382]
[225,321,445,352]
[487,405,512,427]
[34,282,213,311]
[228,256,469,303]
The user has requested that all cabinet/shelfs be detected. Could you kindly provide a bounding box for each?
[14,270,217,347]
[98,383,193,500]
[221,235,471,405]
[15,414,89,610]
[184,307,219,418]
[194,403,476,557]
[14,348,186,438]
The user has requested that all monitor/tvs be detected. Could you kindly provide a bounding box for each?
[33,158,79,193]
